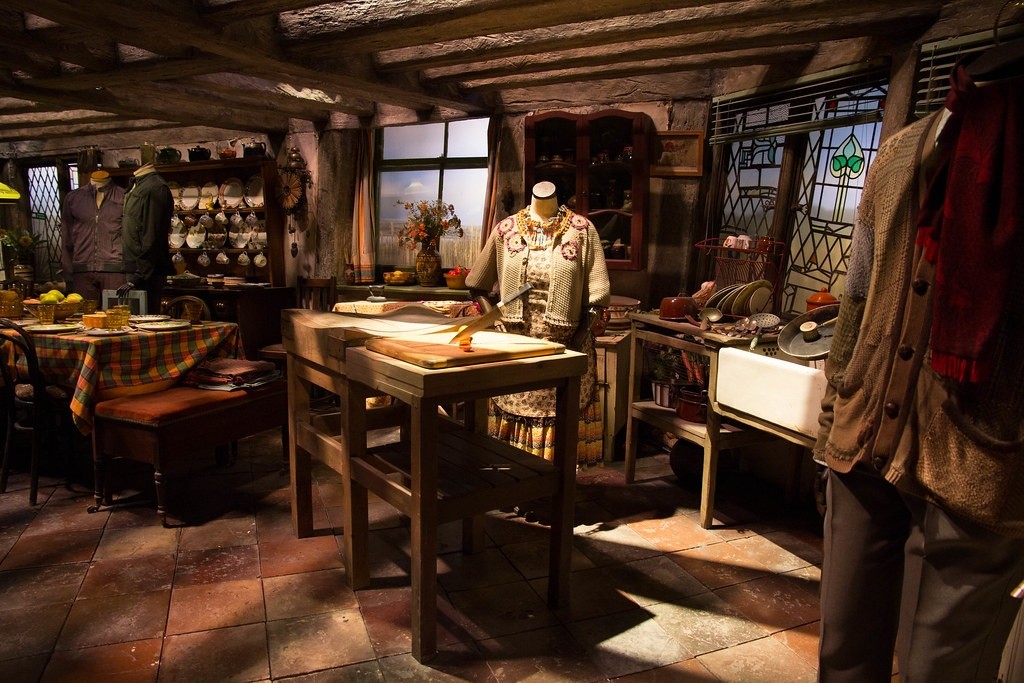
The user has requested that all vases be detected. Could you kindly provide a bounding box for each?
[14,260,34,290]
[415,237,442,287]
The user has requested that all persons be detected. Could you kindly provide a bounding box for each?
[116,162,173,316]
[812,108,1024,683]
[465,181,610,462]
[61,171,126,312]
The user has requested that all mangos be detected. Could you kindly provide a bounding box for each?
[40,290,83,304]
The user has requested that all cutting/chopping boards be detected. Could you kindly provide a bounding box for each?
[364,331,565,369]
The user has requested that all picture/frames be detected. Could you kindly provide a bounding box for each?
[649,130,703,177]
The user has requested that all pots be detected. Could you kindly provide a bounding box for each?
[676,386,708,424]
[659,296,701,327]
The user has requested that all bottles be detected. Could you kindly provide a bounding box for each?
[592,146,632,164]
[539,149,575,168]
[624,189,632,209]
[806,287,837,312]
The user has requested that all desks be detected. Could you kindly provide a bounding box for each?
[0,314,246,506]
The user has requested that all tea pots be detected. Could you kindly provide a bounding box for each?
[118,138,266,167]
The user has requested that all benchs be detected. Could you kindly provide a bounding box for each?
[94,384,289,528]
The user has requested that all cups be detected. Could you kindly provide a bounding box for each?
[38,300,130,331]
[172,251,266,267]
[723,234,752,249]
[171,212,259,227]
[185,302,204,321]
[758,235,774,253]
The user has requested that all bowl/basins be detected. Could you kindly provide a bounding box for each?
[212,282,224,288]
[168,278,202,287]
[22,301,85,319]
[383,272,416,286]
[444,276,468,289]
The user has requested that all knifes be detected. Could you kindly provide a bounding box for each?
[448,281,535,345]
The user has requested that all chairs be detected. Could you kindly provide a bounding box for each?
[0,318,76,506]
[257,275,336,370]
[161,295,211,321]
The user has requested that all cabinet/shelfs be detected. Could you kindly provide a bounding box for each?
[695,236,786,319]
[91,155,296,371]
[595,313,788,530]
[525,109,652,271]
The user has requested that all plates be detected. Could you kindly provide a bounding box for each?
[167,220,265,249]
[224,283,271,288]
[704,278,774,317]
[206,275,245,284]
[168,176,264,208]
[0,311,191,336]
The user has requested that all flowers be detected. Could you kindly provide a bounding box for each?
[0,225,48,267]
[390,199,463,250]
